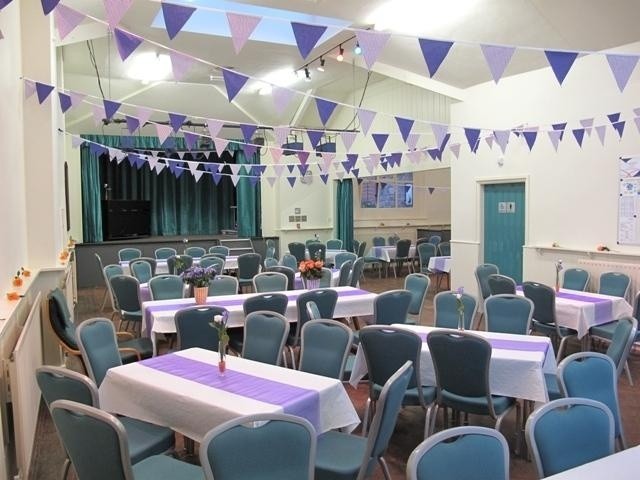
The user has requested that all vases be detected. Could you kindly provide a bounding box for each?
[217,341,226,376]
[457,310,465,331]
[194,286,208,305]
[556,277,559,293]
[173,265,178,275]
[303,277,320,290]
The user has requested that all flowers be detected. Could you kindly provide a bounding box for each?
[555,260,561,281]
[453,287,464,313]
[314,233,320,241]
[208,309,229,361]
[189,266,216,288]
[299,260,323,280]
[172,255,187,270]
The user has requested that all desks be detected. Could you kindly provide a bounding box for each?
[106,346,344,457]
[514,284,624,315]
[361,323,551,402]
[520,439,640,480]
[121,255,240,277]
[428,256,451,291]
[371,246,417,280]
[140,267,357,302]
[286,249,347,265]
[141,286,378,358]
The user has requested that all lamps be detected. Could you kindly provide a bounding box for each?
[304,66,313,83]
[317,58,326,72]
[337,48,345,61]
[355,41,361,55]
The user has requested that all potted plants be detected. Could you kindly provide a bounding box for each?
[14,272,24,288]
[20,267,31,276]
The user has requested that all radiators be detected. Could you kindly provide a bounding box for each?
[1,293,44,479]
[577,259,640,310]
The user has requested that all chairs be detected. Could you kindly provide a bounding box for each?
[386,239,412,279]
[176,306,228,356]
[586,291,640,385]
[264,258,277,266]
[388,236,401,246]
[486,273,516,300]
[334,253,358,270]
[49,398,207,480]
[358,242,381,282]
[306,301,357,372]
[299,319,353,383]
[417,243,441,292]
[288,242,305,262]
[313,360,414,479]
[199,413,316,480]
[439,242,450,257]
[434,290,478,331]
[429,236,441,246]
[42,288,158,377]
[208,245,230,256]
[337,259,353,286]
[184,247,205,257]
[237,253,261,293]
[351,257,365,287]
[264,266,295,291]
[148,274,184,300]
[563,268,590,292]
[75,317,124,390]
[253,272,288,294]
[94,253,109,312]
[103,263,125,319]
[556,351,626,451]
[131,262,152,283]
[475,263,501,329]
[358,325,437,443]
[199,258,224,276]
[293,289,338,346]
[405,426,509,480]
[167,256,193,274]
[307,244,326,261]
[372,237,386,247]
[306,240,322,249]
[483,295,535,336]
[118,248,141,261]
[109,275,142,338]
[404,272,431,324]
[326,239,343,249]
[597,272,631,297]
[544,315,638,401]
[351,289,413,350]
[428,328,522,459]
[240,310,290,367]
[302,268,332,290]
[524,397,615,480]
[282,254,298,272]
[34,367,174,480]
[522,281,584,365]
[189,275,239,298]
[265,239,277,258]
[350,239,360,254]
[410,238,428,273]
[154,247,176,259]
